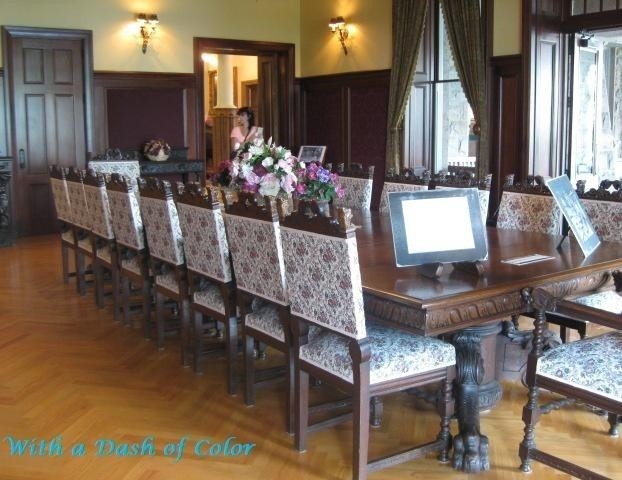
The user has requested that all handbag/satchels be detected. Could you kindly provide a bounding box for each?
[146,140,169,161]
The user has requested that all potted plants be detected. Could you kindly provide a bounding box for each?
[140,136,172,163]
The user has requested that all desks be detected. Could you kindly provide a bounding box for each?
[86,143,204,183]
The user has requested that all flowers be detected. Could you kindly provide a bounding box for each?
[220,133,346,205]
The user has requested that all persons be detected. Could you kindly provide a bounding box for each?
[229,106,258,161]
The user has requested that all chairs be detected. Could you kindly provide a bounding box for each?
[43,165,622,479]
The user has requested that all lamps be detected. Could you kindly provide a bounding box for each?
[326,17,354,58]
[130,10,160,57]
